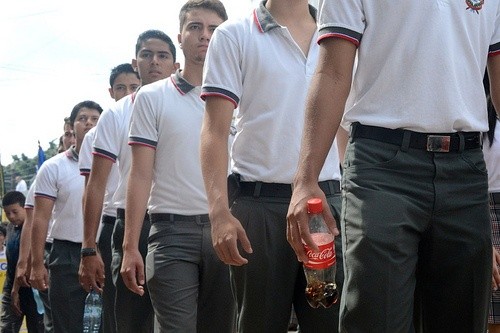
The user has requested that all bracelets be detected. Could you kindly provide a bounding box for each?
[81,247,96,256]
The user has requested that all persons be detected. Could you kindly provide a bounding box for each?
[0,0,500,333]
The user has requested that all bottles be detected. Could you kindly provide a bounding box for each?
[83,289,103,333]
[32,288,45,314]
[302,198,339,308]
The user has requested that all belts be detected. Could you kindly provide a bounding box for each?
[151,212,209,225]
[238,179,341,197]
[102,214,116,224]
[116,208,149,219]
[350,123,484,153]
